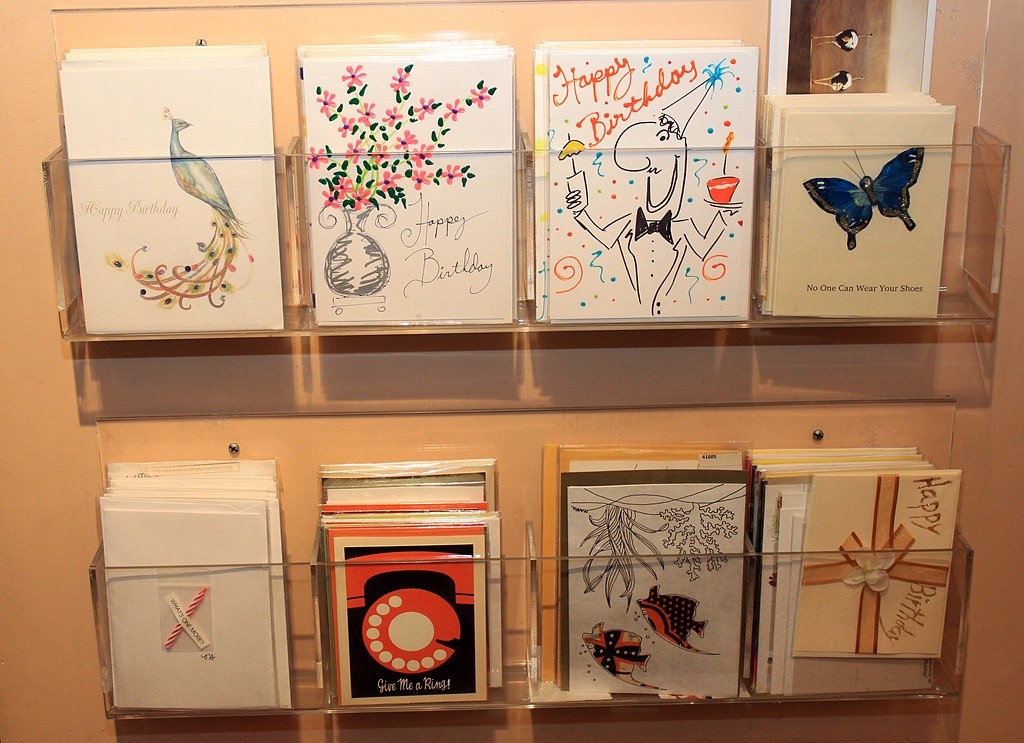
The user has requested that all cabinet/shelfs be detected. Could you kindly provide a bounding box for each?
[41,1,1012,720]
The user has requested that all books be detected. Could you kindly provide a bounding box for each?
[60,40,957,336]
[98,447,963,710]
